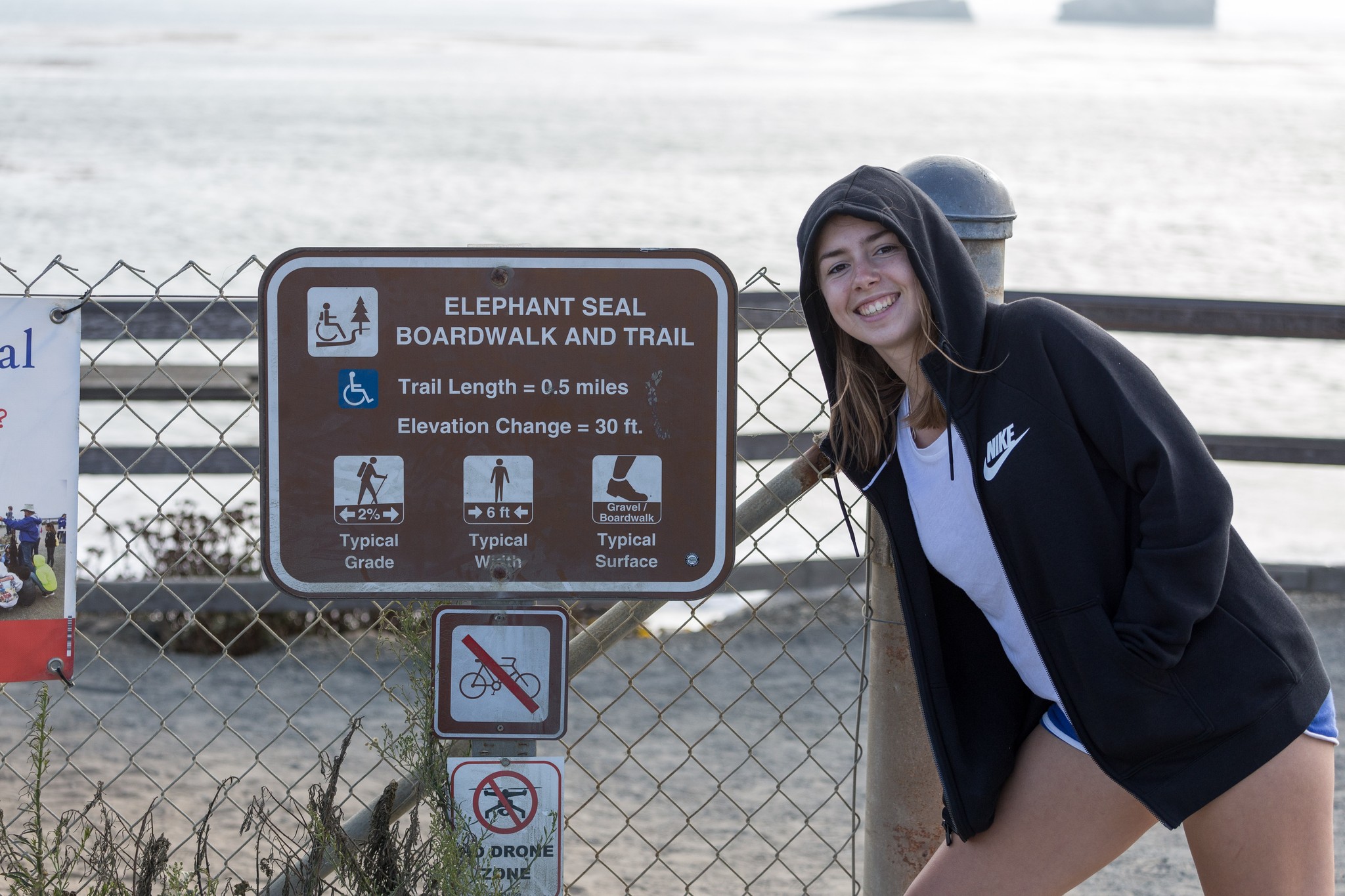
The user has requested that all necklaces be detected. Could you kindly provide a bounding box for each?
[907,386,917,440]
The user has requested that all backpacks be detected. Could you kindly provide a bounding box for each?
[54,535,60,546]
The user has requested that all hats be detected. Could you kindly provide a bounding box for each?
[20,504,35,512]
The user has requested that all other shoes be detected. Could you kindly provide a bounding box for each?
[42,591,55,597]
[17,600,28,608]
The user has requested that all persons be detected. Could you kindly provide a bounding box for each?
[0,504,67,612]
[797,164,1338,896]
